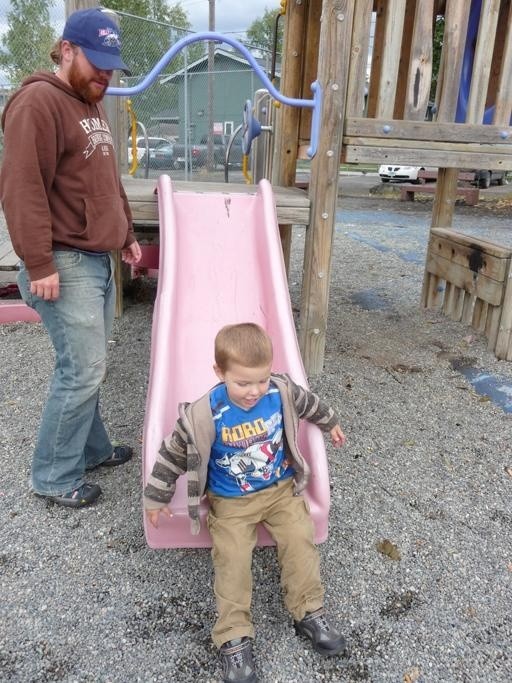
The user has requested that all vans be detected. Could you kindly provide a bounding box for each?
[127,136,172,165]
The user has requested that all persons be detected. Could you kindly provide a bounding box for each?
[144,321,350,683]
[2,5,143,509]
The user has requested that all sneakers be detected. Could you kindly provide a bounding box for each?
[294,606,346,655]
[85,444,133,471]
[220,635,257,682]
[32,482,102,507]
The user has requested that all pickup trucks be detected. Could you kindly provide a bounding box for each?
[172,132,244,170]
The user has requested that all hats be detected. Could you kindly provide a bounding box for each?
[59,7,134,81]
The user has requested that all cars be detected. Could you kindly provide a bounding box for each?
[378,165,440,184]
[164,135,180,142]
[143,143,174,169]
[458,169,508,189]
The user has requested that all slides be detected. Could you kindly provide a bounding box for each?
[140,175,329,547]
